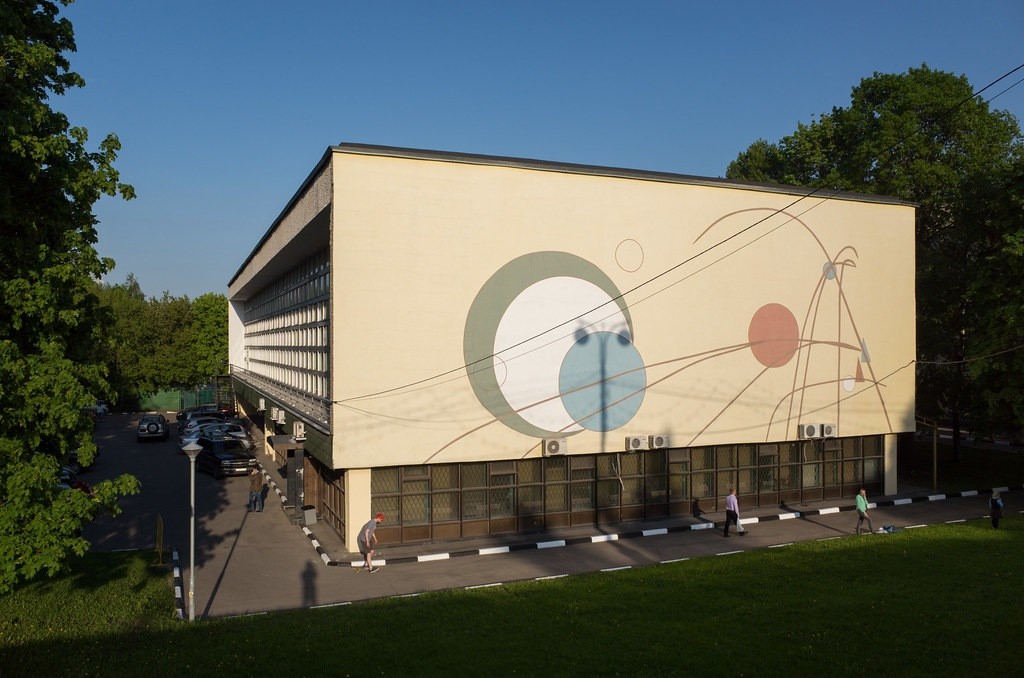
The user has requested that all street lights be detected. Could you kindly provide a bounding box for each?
[181,442,204,622]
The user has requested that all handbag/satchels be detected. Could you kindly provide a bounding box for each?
[875,527,888,534]
[736,516,744,532]
[884,525,897,533]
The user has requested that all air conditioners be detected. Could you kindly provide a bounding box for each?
[292,421,305,438]
[271,406,279,419]
[541,439,567,456]
[820,424,837,437]
[799,423,820,439]
[625,437,648,450]
[258,398,265,409]
[649,434,669,448]
[276,410,285,422]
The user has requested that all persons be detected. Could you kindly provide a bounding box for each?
[247,465,263,512]
[97,403,104,421]
[357,513,384,573]
[989,491,1003,528]
[855,488,877,534]
[723,488,748,537]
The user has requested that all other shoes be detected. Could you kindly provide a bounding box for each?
[724,534,731,537]
[368,567,379,574]
[871,532,877,534]
[256,510,263,512]
[247,508,254,512]
[857,530,861,534]
[740,531,748,536]
[363,564,377,569]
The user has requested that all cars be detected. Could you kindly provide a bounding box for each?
[176,402,252,480]
[57,467,91,497]
[82,402,110,416]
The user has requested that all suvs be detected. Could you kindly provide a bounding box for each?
[135,413,170,442]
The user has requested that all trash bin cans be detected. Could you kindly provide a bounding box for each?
[300,505,317,526]
